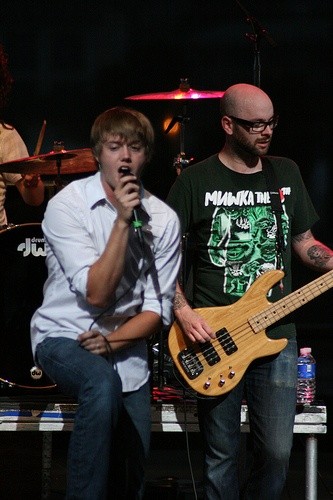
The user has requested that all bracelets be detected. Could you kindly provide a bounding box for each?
[23,176,39,187]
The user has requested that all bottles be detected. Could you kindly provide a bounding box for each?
[296,347,316,406]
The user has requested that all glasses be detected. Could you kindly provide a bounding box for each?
[230,116,280,132]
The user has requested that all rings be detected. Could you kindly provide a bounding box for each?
[97,343,100,349]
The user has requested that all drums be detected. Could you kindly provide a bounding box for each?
[0,223,56,390]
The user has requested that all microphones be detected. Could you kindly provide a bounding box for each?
[121,173,145,243]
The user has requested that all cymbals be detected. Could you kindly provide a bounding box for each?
[0,146,98,175]
[125,91,225,103]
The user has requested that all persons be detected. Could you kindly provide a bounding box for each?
[31,107,182,500]
[167,83,333,500]
[0,45,44,230]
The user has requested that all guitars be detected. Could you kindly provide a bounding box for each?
[166,269,333,400]
